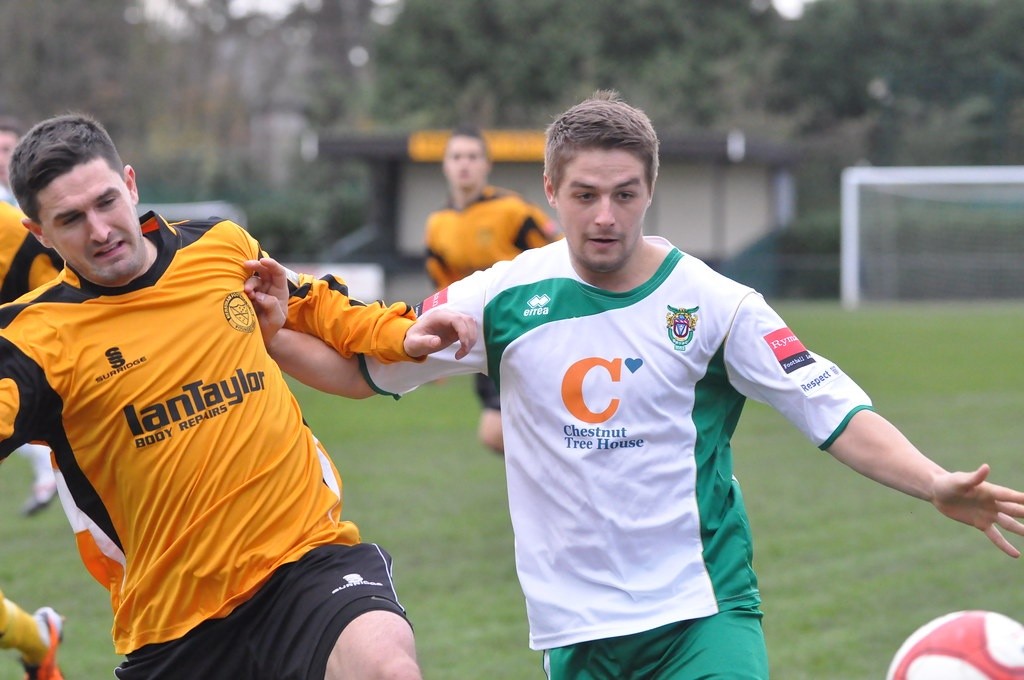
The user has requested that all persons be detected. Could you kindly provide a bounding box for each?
[0,201,69,678]
[0,111,483,680]
[2,121,66,520]
[423,126,568,457]
[240,86,1024,680]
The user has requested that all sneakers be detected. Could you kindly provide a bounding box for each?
[22,479,58,516]
[19,607,66,679]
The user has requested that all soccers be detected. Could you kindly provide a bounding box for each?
[883,609,1024,680]
